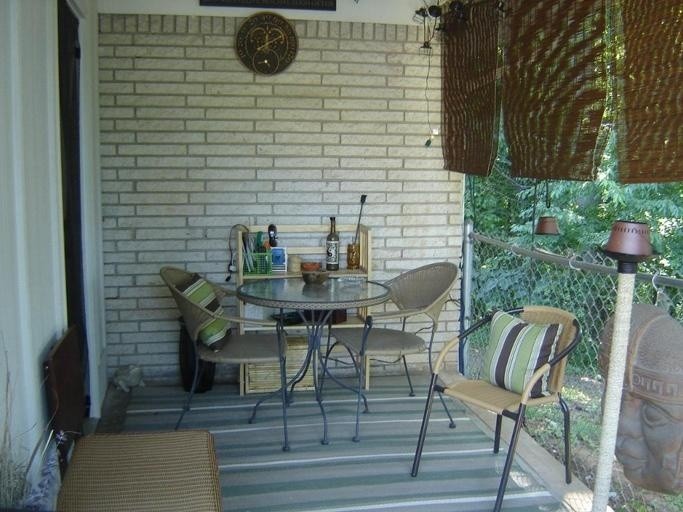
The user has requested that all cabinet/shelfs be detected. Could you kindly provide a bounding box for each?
[233,224,375,397]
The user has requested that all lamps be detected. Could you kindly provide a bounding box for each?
[411,0,469,28]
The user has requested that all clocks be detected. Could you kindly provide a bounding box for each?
[233,10,299,77]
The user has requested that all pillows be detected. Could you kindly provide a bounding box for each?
[483,306,563,400]
[170,271,232,353]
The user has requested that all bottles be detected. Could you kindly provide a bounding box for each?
[327,216,340,270]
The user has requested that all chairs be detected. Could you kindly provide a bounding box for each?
[310,260,459,443]
[41,319,222,511]
[411,302,583,512]
[158,265,292,453]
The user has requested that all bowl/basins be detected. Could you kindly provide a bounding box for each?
[299,270,331,284]
[299,262,320,270]
[302,284,331,299]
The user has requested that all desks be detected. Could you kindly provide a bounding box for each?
[233,276,395,446]
